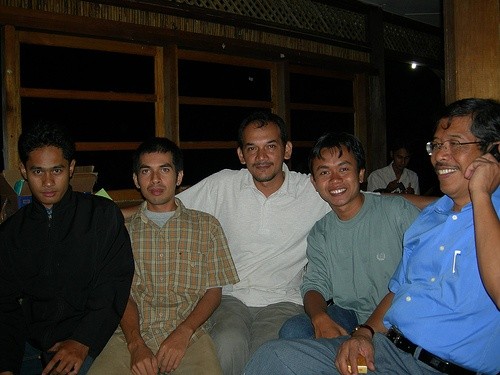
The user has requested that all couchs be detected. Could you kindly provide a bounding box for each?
[102,195,500,372]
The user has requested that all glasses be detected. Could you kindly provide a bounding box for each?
[425,141,485,155]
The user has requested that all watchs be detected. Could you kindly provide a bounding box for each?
[351,323,376,338]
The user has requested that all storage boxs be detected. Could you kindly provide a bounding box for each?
[1,165,98,222]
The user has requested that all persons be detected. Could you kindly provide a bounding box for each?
[88,137,240,375]
[366,144,422,197]
[0,122,135,375]
[244,98,500,374]
[279,132,424,341]
[174,110,447,374]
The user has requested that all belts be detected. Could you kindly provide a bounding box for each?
[386,328,478,375]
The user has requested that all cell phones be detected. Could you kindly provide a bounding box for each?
[488,144,500,163]
